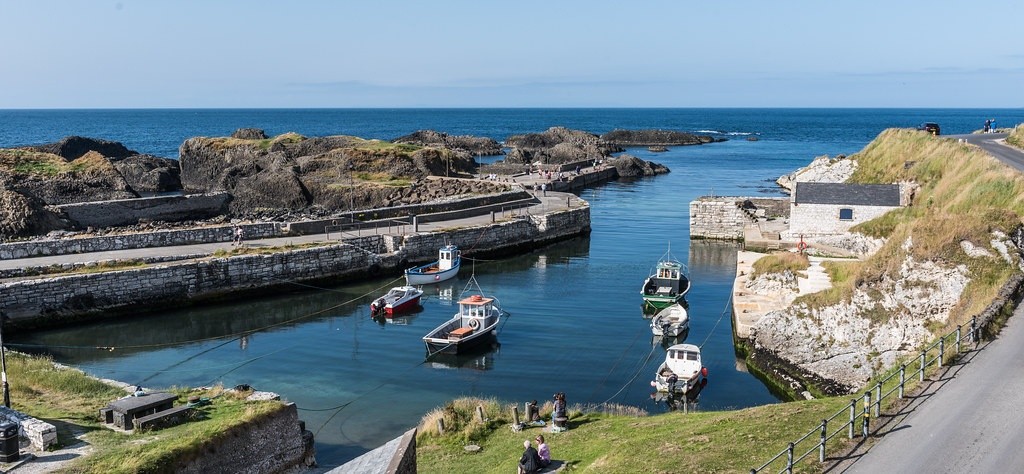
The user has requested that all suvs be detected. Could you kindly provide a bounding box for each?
[917,121,940,136]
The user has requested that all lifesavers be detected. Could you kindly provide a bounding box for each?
[332,220,338,227]
[797,242,807,250]
[467,318,479,329]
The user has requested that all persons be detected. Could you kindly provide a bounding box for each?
[541,183,547,196]
[533,182,539,196]
[552,392,566,427]
[984,119,996,134]
[539,154,606,182]
[535,435,550,471]
[518,440,539,474]
[490,174,516,183]
[231,226,244,245]
[531,400,540,420]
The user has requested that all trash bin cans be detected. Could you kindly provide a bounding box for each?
[0,422,20,463]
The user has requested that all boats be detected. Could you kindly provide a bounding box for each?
[368,284,424,322]
[422,294,504,356]
[402,242,463,287]
[651,303,690,338]
[653,343,703,404]
[640,240,692,309]
